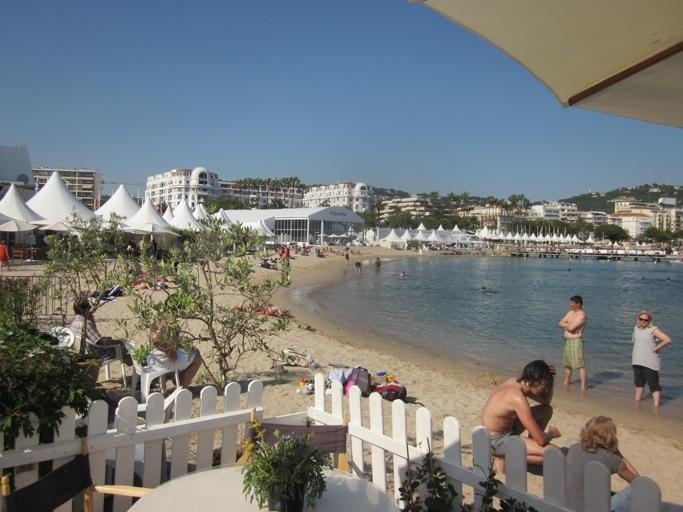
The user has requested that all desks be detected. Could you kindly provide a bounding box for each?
[125,464,399,512]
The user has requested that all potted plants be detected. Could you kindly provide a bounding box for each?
[238,418,333,512]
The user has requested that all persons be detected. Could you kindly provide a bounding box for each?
[563,414,640,511]
[82,274,168,298]
[481,360,561,475]
[632,310,672,406]
[262,241,381,273]
[0,240,10,276]
[73,298,149,367]
[558,294,586,393]
[28,233,37,247]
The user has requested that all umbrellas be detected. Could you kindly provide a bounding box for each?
[428,1,682,129]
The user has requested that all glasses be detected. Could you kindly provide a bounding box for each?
[639,316,649,322]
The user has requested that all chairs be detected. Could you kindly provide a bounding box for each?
[234,407,353,471]
[51,325,179,403]
[0,436,153,512]
